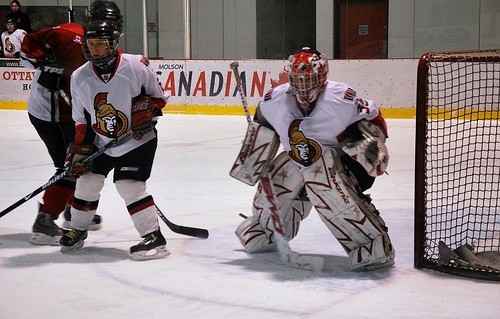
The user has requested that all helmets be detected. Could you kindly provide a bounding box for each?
[5,16,17,34]
[81,20,121,68]
[84,0,125,38]
[283,46,329,110]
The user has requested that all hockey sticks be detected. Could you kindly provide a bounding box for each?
[60,88,210,239]
[0,128,132,219]
[229,61,325,272]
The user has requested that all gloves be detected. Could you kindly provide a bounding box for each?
[131,96,155,140]
[64,142,93,179]
[38,56,67,93]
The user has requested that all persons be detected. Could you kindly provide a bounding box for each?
[229,47,395,272]
[60,21,171,254]
[20,0,123,237]
[0,0,32,57]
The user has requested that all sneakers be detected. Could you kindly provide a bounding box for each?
[28,213,64,245]
[59,228,87,252]
[62,206,102,230]
[131,226,171,261]
